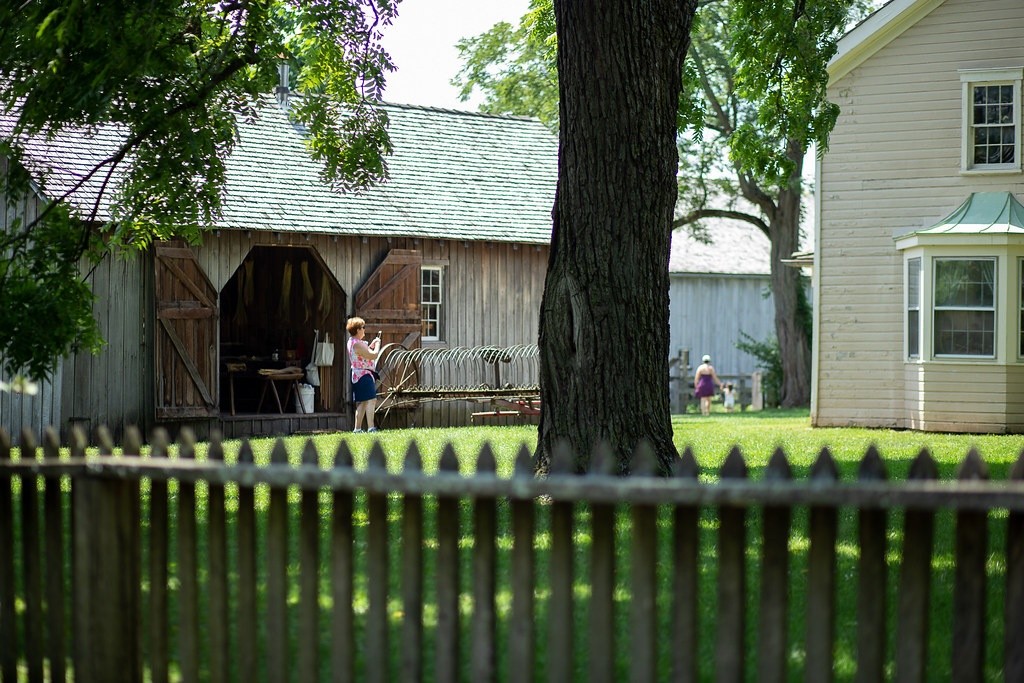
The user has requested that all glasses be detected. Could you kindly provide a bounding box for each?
[360,325,365,329]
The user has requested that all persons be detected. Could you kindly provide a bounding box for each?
[720,383,737,414]
[694,354,721,416]
[346,316,382,434]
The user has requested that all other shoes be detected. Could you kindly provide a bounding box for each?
[354,427,377,433]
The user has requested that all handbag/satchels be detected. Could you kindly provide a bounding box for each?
[367,369,382,383]
[306,363,321,387]
[314,332,335,367]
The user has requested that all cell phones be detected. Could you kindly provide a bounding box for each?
[377,331,382,339]
